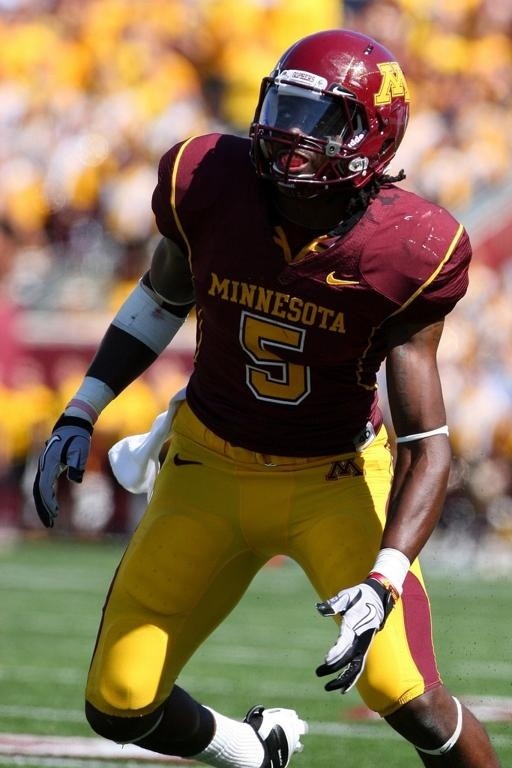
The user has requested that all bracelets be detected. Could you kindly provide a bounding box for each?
[368,547,411,598]
[367,571,400,605]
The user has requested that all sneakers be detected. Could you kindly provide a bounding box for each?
[243,704,310,767]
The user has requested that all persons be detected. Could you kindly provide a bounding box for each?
[33,29,501,767]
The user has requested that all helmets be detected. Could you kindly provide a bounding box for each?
[242,29,412,215]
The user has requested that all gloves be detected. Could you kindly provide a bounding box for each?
[32,413,95,530]
[315,573,399,696]
